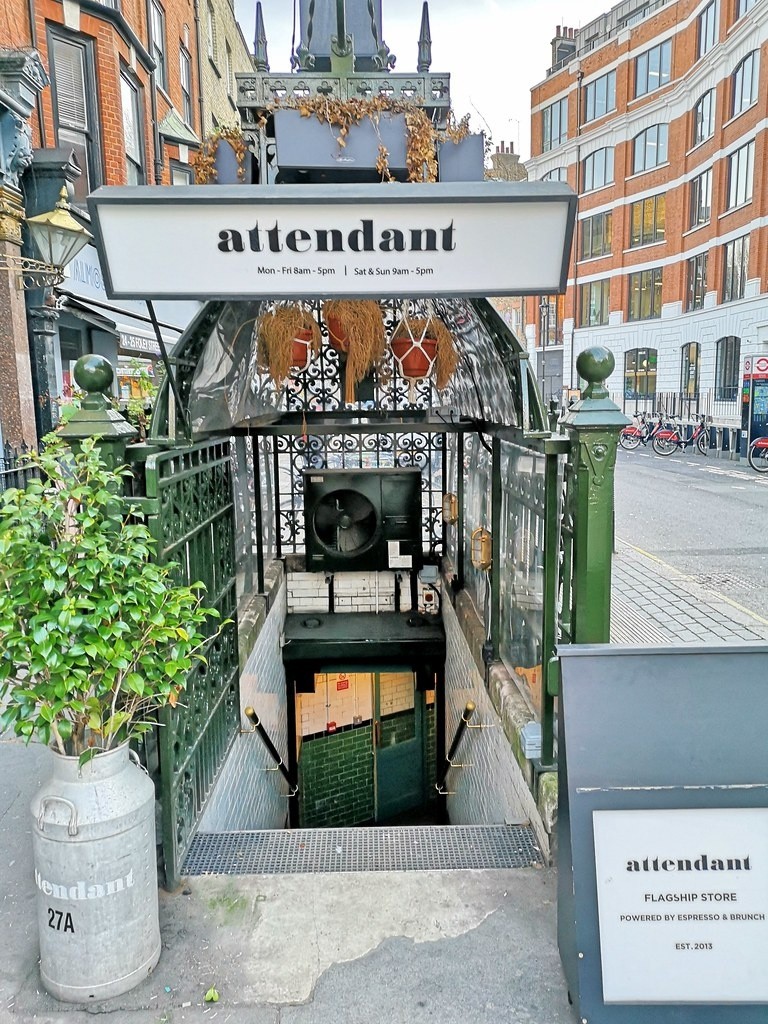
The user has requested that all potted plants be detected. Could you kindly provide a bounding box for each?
[256,303,321,391]
[321,299,388,404]
[0,426,236,1002]
[392,316,463,389]
[436,108,485,182]
[189,124,251,184]
[490,140,520,170]
[255,93,439,183]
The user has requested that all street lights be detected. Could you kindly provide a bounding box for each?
[537,296,550,405]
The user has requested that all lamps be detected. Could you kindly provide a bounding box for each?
[441,492,458,525]
[471,527,493,571]
[0,185,95,290]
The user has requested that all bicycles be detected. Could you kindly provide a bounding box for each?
[615,409,676,451]
[650,412,719,457]
[746,421,768,473]
